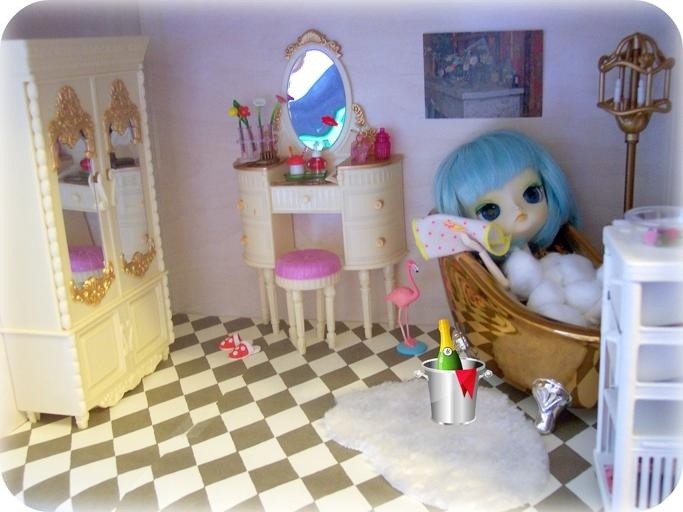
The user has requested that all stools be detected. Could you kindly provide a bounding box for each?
[274,249,342,355]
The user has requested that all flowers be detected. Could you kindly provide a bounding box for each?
[228,95,293,151]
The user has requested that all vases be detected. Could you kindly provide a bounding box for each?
[237,126,274,158]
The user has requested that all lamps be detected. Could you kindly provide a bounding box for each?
[597,32,674,216]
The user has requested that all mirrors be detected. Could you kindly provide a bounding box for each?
[46,80,157,308]
[281,28,354,161]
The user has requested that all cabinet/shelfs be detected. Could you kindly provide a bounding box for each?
[231,154,406,337]
[590,225,682,510]
[0,33,176,431]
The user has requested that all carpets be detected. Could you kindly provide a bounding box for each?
[322,378,550,511]
